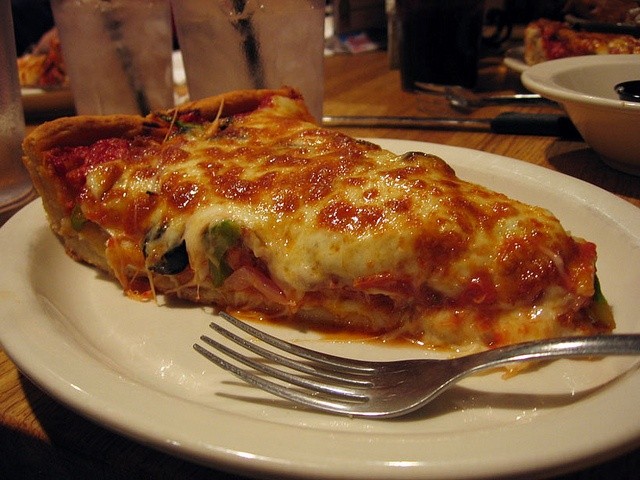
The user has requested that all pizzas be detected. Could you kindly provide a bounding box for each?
[22,86,616,380]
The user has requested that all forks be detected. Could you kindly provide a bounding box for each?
[192,312,640,420]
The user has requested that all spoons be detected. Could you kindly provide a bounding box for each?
[445,90,557,115]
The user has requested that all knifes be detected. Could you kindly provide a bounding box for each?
[322,112,579,139]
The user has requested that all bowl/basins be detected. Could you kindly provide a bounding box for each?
[522,55,640,177]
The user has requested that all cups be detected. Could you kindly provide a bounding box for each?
[49,1,173,116]
[0,0,42,225]
[172,0,325,127]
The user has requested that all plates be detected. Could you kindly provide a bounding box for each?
[502,45,530,72]
[0,137,640,480]
